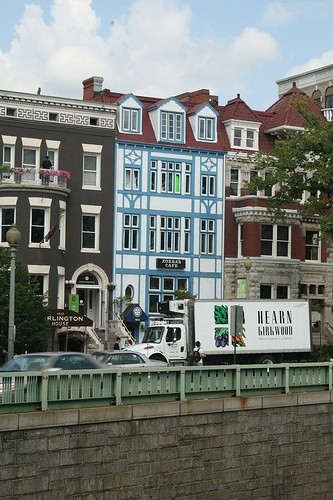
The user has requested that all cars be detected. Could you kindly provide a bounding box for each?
[89,350,174,383]
[0,351,113,398]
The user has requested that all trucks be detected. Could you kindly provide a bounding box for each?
[129,299,311,377]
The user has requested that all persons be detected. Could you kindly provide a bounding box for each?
[41,156,52,185]
[193,340,207,365]
[114,337,122,349]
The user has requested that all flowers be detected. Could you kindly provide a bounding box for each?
[11,167,71,180]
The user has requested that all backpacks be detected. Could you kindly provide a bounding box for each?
[192,348,201,364]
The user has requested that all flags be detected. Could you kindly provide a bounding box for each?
[45,218,60,242]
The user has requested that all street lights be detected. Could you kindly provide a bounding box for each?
[242,256,253,301]
[6,225,22,358]
